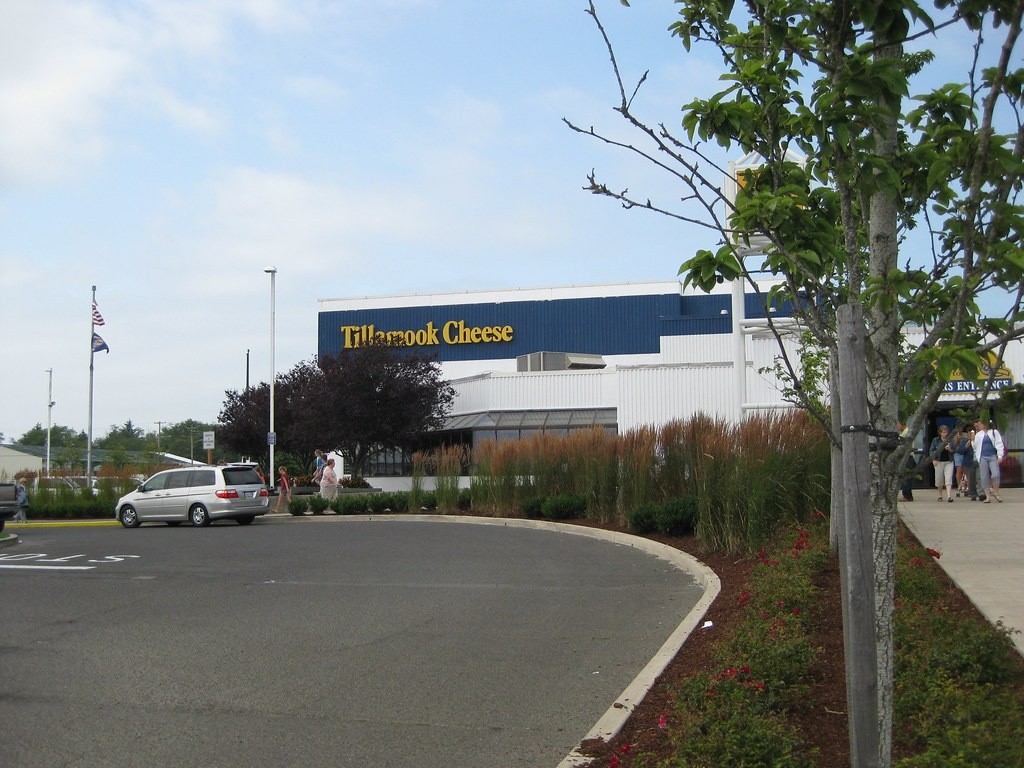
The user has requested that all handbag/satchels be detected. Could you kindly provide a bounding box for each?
[993,429,1008,457]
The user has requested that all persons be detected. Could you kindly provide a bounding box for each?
[13,478,27,523]
[271,466,291,514]
[896,418,913,502]
[217,459,265,484]
[311,449,339,502]
[930,419,1004,503]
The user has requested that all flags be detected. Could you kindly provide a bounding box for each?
[92,299,106,326]
[91,331,110,353]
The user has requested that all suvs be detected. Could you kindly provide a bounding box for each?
[33,476,144,498]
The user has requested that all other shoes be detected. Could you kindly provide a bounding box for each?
[938,496,943,501]
[971,496,976,501]
[948,497,955,502]
[995,493,1003,502]
[979,495,985,501]
[272,509,279,514]
[984,497,992,503]
[898,496,914,502]
[14,515,18,523]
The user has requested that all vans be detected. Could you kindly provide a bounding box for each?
[115,464,269,529]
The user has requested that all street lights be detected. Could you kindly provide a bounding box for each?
[46,367,52,475]
[264,265,278,491]
